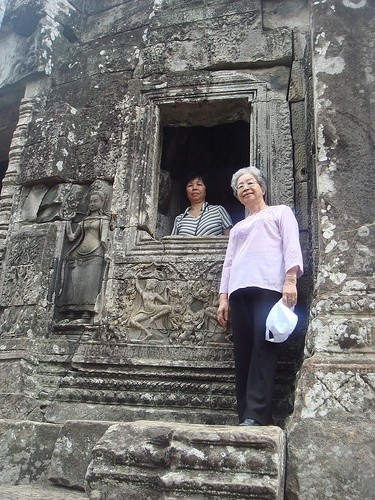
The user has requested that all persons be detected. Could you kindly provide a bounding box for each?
[216,167,305,425]
[203,293,233,326]
[170,172,234,237]
[129,274,172,341]
[57,188,111,325]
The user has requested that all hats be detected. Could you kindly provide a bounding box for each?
[265,296,298,343]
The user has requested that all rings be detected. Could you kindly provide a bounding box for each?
[288,298,292,301]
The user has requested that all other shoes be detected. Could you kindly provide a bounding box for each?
[239,418,260,426]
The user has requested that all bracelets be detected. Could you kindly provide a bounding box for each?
[281,274,297,284]
